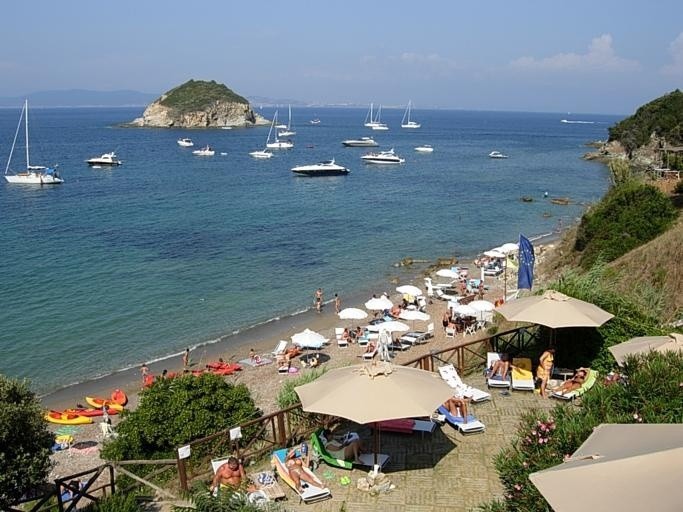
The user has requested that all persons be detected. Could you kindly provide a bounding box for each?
[248,348,264,365]
[322,428,363,464]
[181,347,190,366]
[550,369,586,397]
[443,395,474,425]
[210,455,255,497]
[59,477,83,499]
[203,355,240,376]
[285,446,327,494]
[157,368,167,380]
[487,358,510,381]
[138,363,149,381]
[102,398,111,426]
[313,288,325,314]
[278,345,299,369]
[536,343,556,401]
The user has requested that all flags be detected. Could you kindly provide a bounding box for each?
[518,234,534,290]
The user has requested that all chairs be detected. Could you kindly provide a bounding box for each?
[271,268,503,361]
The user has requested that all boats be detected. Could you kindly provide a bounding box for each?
[86,148,123,170]
[35,385,128,427]
[138,361,242,391]
[172,101,323,163]
[4,98,65,187]
[560,120,568,124]
[343,136,441,168]
[488,149,510,160]
[290,152,354,177]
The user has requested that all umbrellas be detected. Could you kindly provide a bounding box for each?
[528,423,683,512]
[290,328,324,367]
[335,242,519,359]
[290,358,457,465]
[607,332,683,368]
[490,289,616,361]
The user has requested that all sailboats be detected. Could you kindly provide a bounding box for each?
[360,98,424,132]
